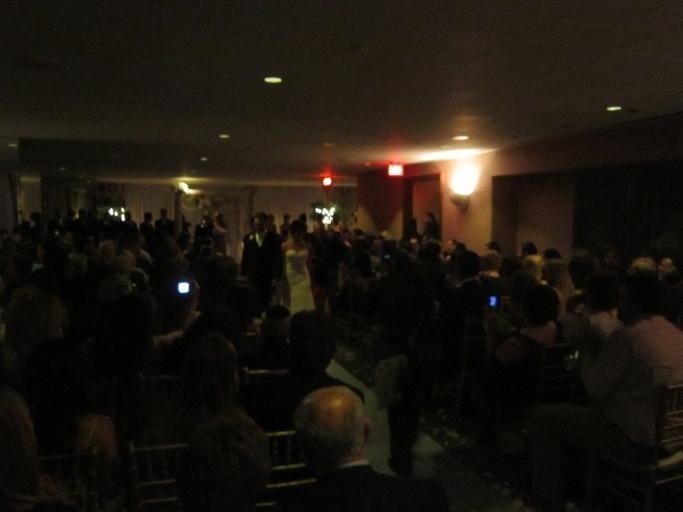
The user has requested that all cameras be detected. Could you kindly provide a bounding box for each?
[175,280,193,298]
[489,296,498,308]
